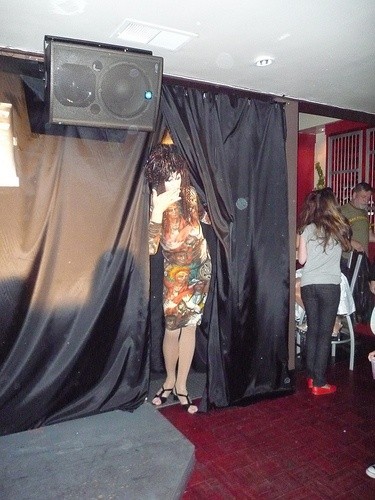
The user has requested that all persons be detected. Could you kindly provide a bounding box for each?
[337,182,375,334]
[297,187,354,395]
[364,351,375,479]
[144,143,221,409]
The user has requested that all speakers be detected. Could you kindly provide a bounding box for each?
[42,35,164,132]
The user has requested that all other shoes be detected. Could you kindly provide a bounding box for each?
[366,465,375,478]
[313,384,337,394]
[307,378,314,388]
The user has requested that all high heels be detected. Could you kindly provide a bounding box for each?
[173,386,198,414]
[151,386,172,405]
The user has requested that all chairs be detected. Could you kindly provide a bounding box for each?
[332,250,363,371]
